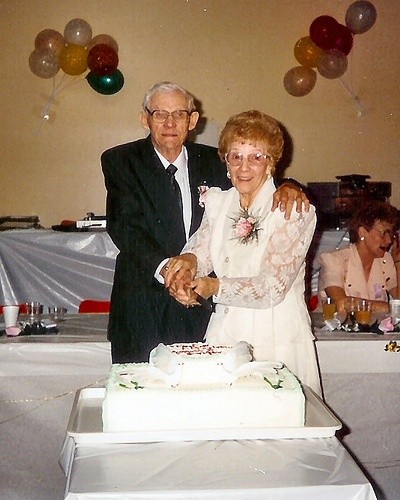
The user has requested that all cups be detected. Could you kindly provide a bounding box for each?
[344,298,359,326]
[2,306,20,328]
[321,298,337,324]
[48,306,64,324]
[356,299,372,331]
[25,302,40,325]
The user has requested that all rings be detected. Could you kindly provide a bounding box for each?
[165,266,169,272]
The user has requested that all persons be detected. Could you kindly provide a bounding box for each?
[316,202,400,323]
[100,81,310,364]
[161,110,325,406]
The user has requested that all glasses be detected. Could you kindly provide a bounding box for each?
[374,226,396,240]
[224,152,270,165]
[145,105,192,123]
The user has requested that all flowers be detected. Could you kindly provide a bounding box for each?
[198,186,209,207]
[226,206,268,247]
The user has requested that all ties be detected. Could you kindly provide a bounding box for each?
[166,164,186,246]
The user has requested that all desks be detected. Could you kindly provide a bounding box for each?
[0,228,400,314]
[0,312,400,500]
[59,436,379,500]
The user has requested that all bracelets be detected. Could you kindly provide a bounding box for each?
[276,178,304,191]
[394,260,400,264]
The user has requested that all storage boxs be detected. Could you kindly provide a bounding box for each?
[308,181,391,218]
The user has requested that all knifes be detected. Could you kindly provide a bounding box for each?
[183,282,214,313]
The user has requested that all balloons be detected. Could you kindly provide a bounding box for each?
[85,68,125,96]
[64,18,93,48]
[87,43,119,77]
[59,45,88,76]
[309,15,338,50]
[333,23,353,57]
[293,36,323,67]
[34,29,66,58]
[28,47,61,80]
[86,34,119,55]
[283,66,317,98]
[316,47,348,79]
[345,0,377,34]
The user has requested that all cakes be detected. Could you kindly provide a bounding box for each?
[102,342,306,433]
